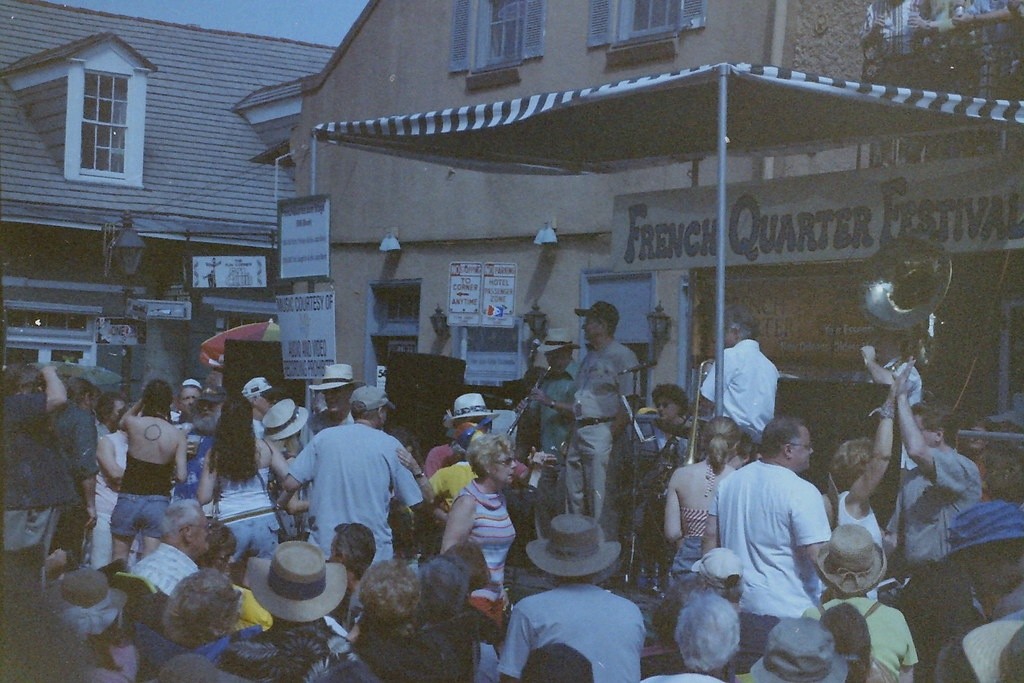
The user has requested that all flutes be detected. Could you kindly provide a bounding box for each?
[507,365,552,436]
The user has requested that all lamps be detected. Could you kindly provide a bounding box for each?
[429,303,453,339]
[101,209,147,280]
[523,299,548,340]
[647,299,671,345]
[533,217,559,245]
[378,227,401,252]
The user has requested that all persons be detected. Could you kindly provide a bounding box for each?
[1,360,1024,683]
[686,303,781,462]
[857,0,1024,171]
[512,332,582,588]
[563,299,642,590]
[846,324,925,490]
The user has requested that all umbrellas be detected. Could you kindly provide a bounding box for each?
[199,316,282,368]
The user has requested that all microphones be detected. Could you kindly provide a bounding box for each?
[527,337,541,364]
[616,360,657,377]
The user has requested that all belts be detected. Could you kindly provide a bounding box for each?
[578,415,616,425]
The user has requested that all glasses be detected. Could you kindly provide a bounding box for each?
[493,457,517,466]
[179,522,212,532]
[320,386,350,394]
[786,440,815,451]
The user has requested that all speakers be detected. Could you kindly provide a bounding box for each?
[955,430,1023,508]
[382,352,468,468]
[774,378,899,531]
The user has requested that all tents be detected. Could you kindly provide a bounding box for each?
[304,60,1024,420]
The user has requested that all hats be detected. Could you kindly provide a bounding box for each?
[182,378,203,392]
[240,377,272,400]
[932,497,1024,572]
[441,392,501,428]
[246,540,350,623]
[348,384,396,412]
[308,363,362,391]
[959,607,1024,683]
[748,616,850,683]
[261,398,309,442]
[196,386,227,403]
[574,301,620,326]
[814,524,889,597]
[38,567,129,637]
[537,328,582,353]
[690,547,745,591]
[525,513,622,578]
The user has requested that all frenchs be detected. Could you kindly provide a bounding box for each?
[847,234,957,377]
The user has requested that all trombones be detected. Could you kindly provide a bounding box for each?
[681,358,714,467]
